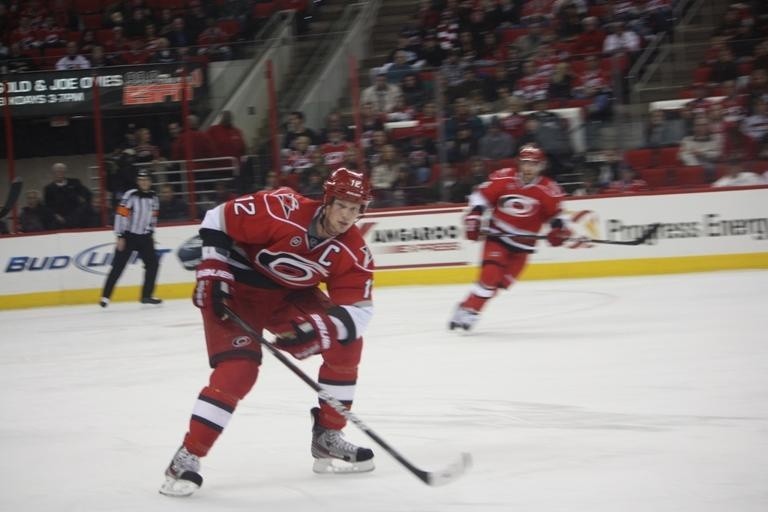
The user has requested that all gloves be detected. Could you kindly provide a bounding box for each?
[273,311,338,358]
[549,228,569,246]
[465,210,483,240]
[192,261,237,322]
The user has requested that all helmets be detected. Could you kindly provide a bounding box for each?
[519,146,544,163]
[323,168,374,213]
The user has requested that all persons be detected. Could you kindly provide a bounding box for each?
[622,2,765,187]
[103,108,246,218]
[261,109,358,199]
[1,2,312,72]
[155,168,375,498]
[440,143,575,337]
[97,169,161,309]
[359,2,675,207]
[43,161,93,228]
[20,189,63,234]
[80,196,114,228]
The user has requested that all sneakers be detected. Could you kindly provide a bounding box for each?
[164,449,203,487]
[140,297,160,305]
[311,429,373,461]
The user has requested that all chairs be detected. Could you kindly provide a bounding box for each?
[1,0,284,73]
[284,0,768,213]
